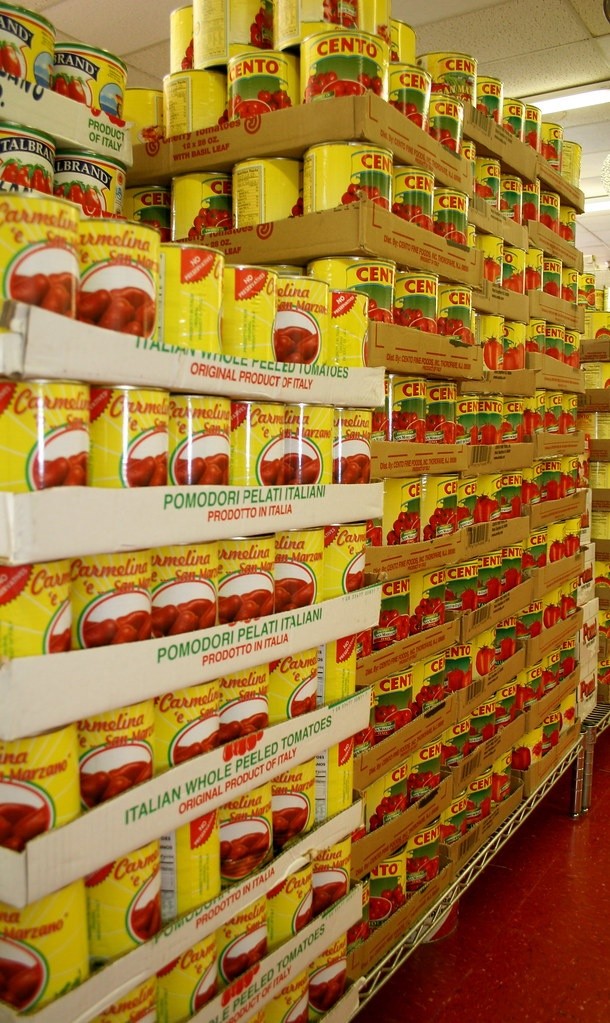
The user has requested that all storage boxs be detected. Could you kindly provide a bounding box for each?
[0,68,610,1023]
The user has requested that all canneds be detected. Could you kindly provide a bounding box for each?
[0,0,610,1023]
